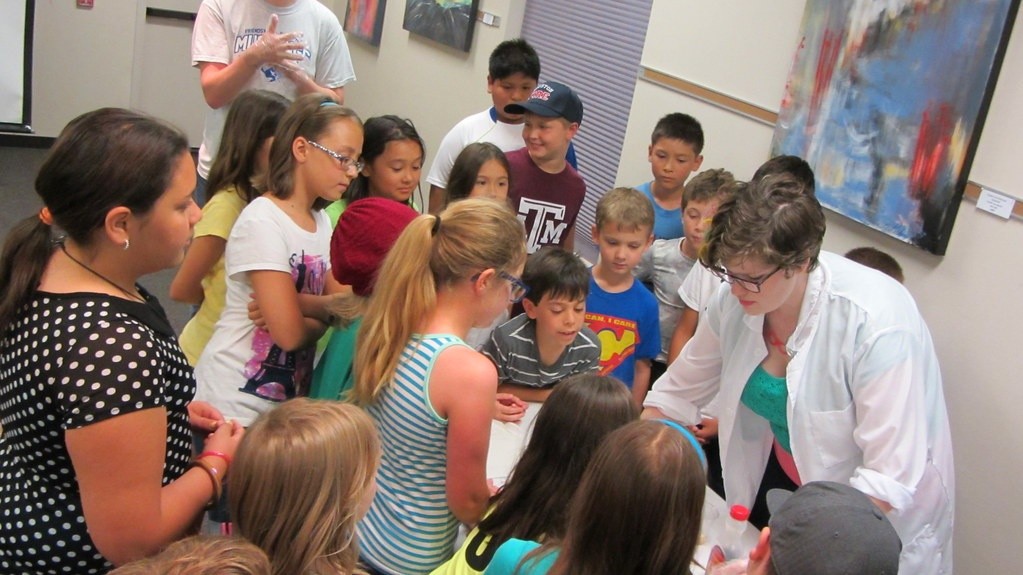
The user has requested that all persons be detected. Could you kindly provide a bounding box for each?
[103,532,272,575]
[224,394,383,575]
[187,1,358,209]
[170,34,958,575]
[0,108,245,575]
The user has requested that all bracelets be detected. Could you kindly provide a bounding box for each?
[186,461,219,510]
[195,449,232,466]
[198,458,223,498]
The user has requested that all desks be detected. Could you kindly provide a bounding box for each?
[487,400,764,575]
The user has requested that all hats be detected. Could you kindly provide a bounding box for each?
[504,81,584,127]
[329,197,423,298]
[766,481,903,575]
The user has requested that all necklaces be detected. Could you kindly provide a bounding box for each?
[59,239,163,322]
[764,322,790,355]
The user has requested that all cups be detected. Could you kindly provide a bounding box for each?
[697,504,719,546]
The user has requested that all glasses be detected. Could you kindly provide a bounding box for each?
[470,268,529,302]
[306,139,364,175]
[705,250,804,294]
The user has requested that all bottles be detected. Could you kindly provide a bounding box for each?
[705,504,750,575]
